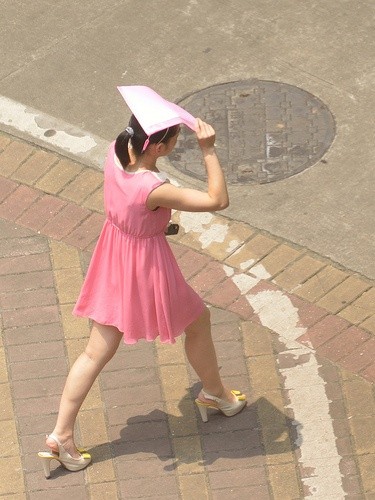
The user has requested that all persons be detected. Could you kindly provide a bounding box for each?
[38,113,246,479]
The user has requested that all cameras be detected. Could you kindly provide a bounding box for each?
[165,224,179,235]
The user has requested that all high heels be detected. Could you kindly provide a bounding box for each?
[194,388,247,423]
[38,434,91,480]
